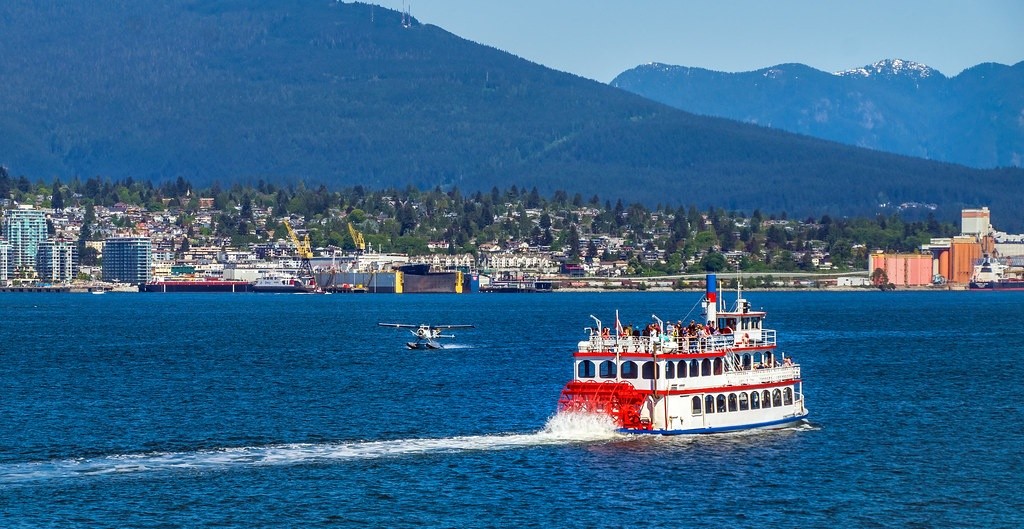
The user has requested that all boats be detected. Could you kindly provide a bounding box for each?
[556,273,810,437]
[250,269,319,293]
[90,285,105,295]
[110,283,140,293]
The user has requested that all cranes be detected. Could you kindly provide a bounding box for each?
[347,222,366,255]
[281,218,313,259]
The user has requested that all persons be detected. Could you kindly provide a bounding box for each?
[704,392,759,414]
[589,319,735,352]
[743,354,791,370]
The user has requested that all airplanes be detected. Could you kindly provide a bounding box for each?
[377,321,476,349]
